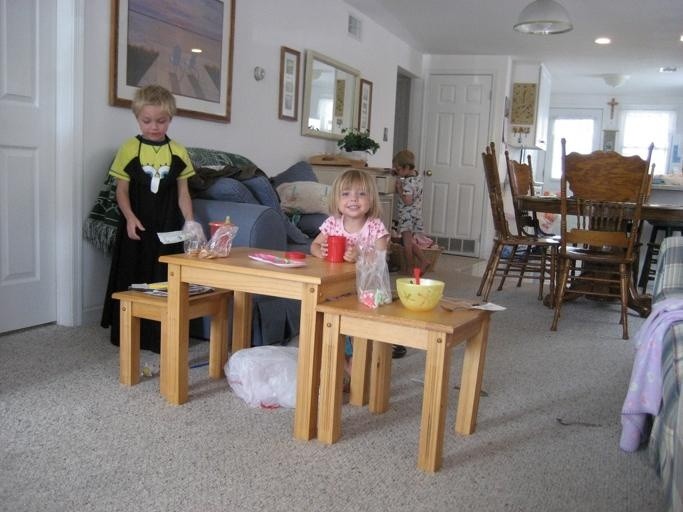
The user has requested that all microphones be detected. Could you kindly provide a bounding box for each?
[112,285,231,407]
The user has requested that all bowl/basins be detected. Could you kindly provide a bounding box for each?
[150,139,161,155]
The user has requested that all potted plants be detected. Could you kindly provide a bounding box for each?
[280,46,300,121]
[359,79,374,139]
[302,49,359,143]
[109,0,233,124]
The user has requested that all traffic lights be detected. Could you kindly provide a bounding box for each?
[244,177,276,212]
[271,163,315,197]
[213,177,260,209]
[278,181,337,216]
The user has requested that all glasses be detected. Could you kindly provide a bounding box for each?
[603,74,630,89]
[508,1,573,34]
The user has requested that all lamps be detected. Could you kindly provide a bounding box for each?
[476,143,563,309]
[553,136,651,339]
[502,145,570,289]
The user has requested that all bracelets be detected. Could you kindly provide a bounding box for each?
[395,277,442,311]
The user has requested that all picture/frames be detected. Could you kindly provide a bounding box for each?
[315,292,495,472]
[158,249,370,440]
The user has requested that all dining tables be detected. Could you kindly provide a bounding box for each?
[337,127,379,166]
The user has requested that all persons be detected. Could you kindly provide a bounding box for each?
[309,168,389,394]
[390,151,433,277]
[100,84,195,353]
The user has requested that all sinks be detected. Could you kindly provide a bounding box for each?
[620,237,681,512]
[80,149,360,353]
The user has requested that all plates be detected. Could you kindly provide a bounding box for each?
[312,164,394,248]
[509,60,549,149]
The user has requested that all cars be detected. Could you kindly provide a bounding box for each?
[324,235,345,263]
[208,220,235,256]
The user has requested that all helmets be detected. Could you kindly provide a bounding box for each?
[248,253,305,269]
